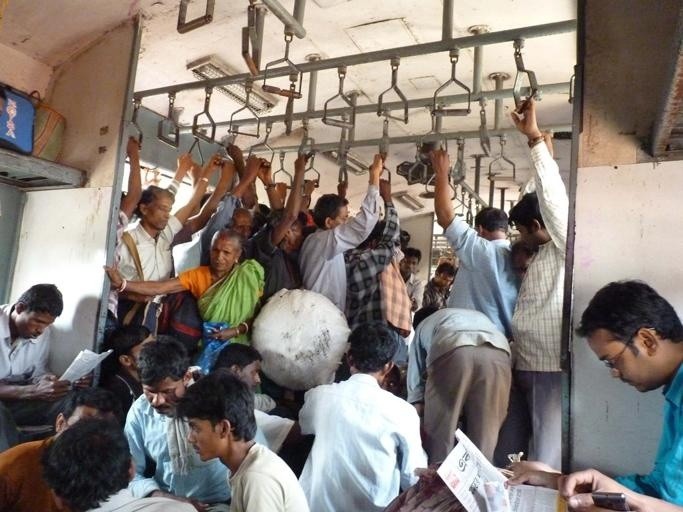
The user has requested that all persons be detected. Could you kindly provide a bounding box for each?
[0,138,531,511]
[508,98,568,467]
[501,280,682,511]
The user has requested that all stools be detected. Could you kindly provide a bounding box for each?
[16,422,56,444]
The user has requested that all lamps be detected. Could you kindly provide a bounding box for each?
[186,55,278,115]
[323,146,370,177]
[392,191,425,212]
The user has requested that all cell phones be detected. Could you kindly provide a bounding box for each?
[592,491,629,511]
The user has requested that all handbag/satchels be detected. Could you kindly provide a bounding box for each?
[33,91,66,161]
[118,298,163,333]
[0,84,34,153]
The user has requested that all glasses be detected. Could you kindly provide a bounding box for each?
[603,326,655,368]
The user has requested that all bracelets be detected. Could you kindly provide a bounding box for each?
[528,136,544,147]
